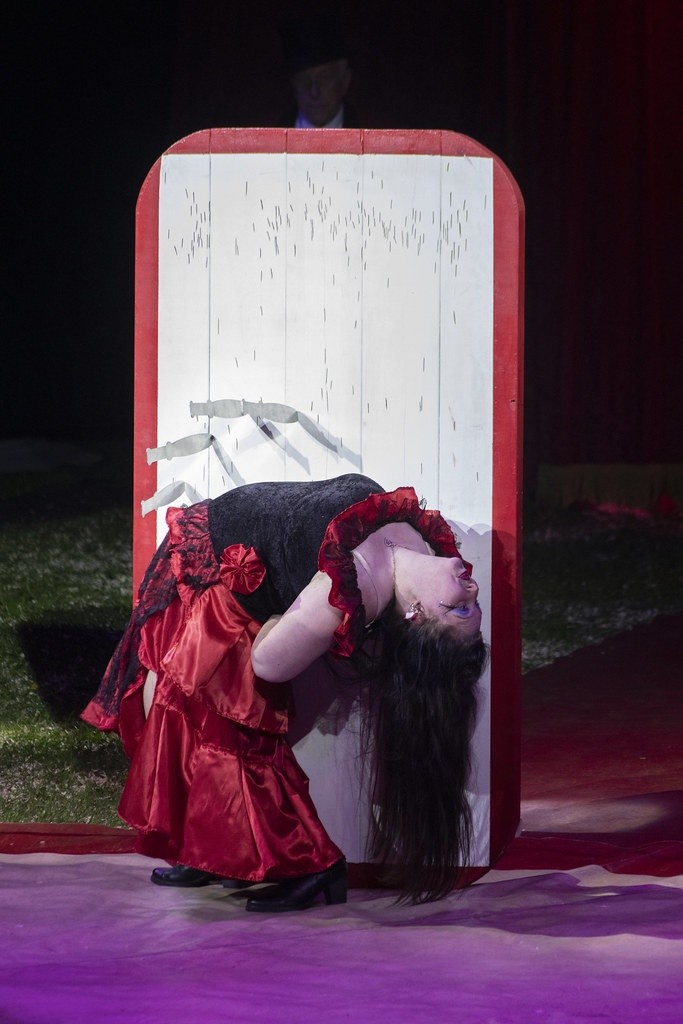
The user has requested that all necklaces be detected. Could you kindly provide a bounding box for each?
[383,537,412,591]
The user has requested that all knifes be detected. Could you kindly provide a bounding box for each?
[145,433,214,465]
[141,481,186,517]
[190,399,299,425]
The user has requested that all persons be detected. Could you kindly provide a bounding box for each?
[80,473,488,913]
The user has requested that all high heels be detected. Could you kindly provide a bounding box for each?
[247,856,348,910]
[151,862,245,889]
